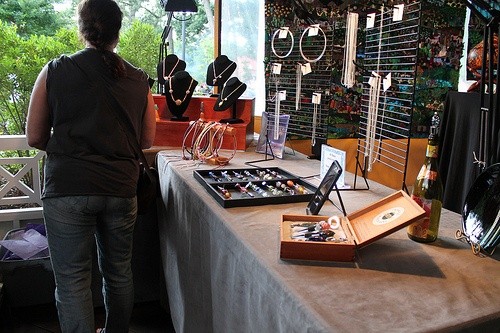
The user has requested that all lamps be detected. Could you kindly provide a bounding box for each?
[157,0,198,95]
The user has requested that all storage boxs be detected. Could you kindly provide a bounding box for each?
[280,190,426,264]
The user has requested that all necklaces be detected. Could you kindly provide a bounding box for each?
[162,58,179,80]
[212,62,233,84]
[219,81,243,107]
[269,13,386,172]
[169,76,193,105]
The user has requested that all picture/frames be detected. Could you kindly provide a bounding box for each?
[306,160,342,216]
[320,144,346,186]
[255,112,290,159]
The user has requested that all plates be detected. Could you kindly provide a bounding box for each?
[461,163,500,251]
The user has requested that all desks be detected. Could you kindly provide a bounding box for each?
[153,138,500,333]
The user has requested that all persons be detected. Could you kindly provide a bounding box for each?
[26,0,157,333]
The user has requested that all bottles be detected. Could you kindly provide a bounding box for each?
[407,138,443,244]
